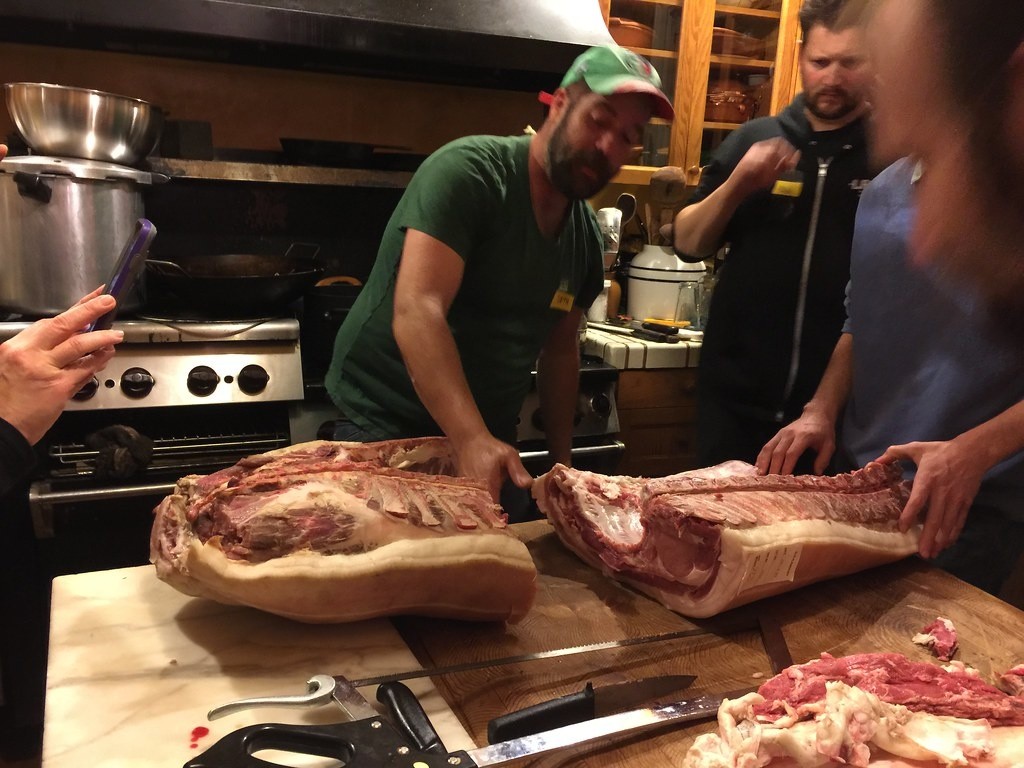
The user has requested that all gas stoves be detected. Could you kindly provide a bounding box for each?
[287,355,622,444]
[0,313,307,406]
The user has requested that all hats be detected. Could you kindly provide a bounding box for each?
[561,44,676,123]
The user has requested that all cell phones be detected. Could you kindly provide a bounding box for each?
[85,217,157,357]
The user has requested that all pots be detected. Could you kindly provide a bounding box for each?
[144,241,326,310]
[0,157,174,317]
[278,136,415,168]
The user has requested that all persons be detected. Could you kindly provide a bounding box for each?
[670,0,887,476]
[321,45,675,517]
[753,153,1024,610]
[833,0,1024,311]
[0,283,123,767]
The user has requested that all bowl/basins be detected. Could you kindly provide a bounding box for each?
[4,82,164,167]
[712,34,765,55]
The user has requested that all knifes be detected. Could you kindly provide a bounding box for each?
[487,675,698,744]
[587,320,689,343]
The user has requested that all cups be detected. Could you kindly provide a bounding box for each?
[588,287,608,323]
[674,282,700,330]
